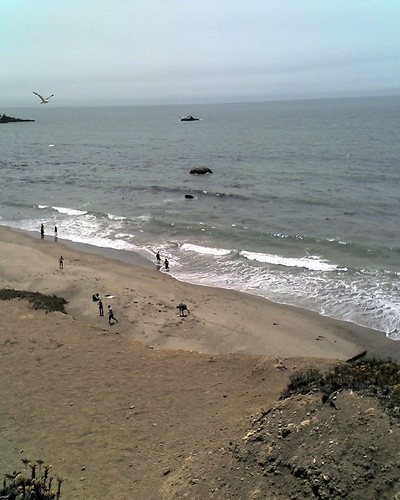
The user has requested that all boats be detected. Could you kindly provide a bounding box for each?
[180,115,199,121]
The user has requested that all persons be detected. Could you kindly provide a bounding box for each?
[55,226,57,232]
[164,259,169,269]
[98,299,104,316]
[93,293,100,302]
[41,224,44,237]
[176,303,190,317]
[156,251,161,264]
[59,256,63,265]
[107,305,118,323]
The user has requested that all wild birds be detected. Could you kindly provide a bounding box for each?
[33,92,54,104]
